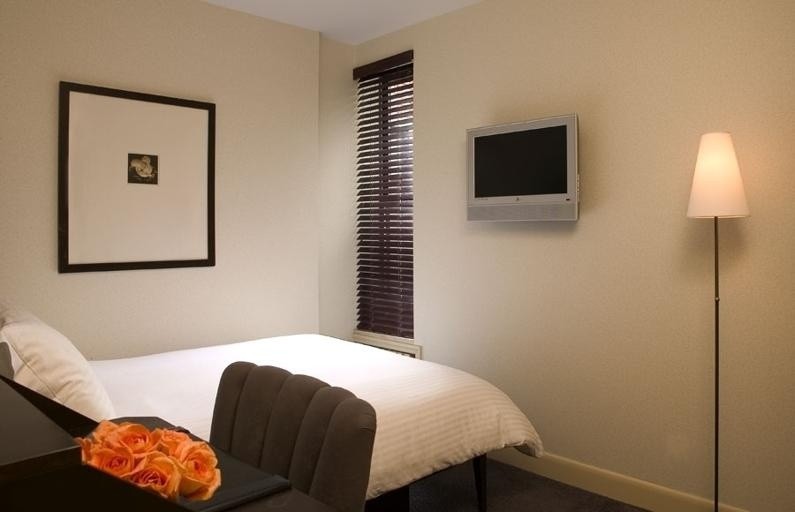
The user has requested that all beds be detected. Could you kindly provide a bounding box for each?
[3,301,544,511]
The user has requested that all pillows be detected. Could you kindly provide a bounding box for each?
[2,306,116,423]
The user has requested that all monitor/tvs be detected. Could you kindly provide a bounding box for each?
[466,111,580,224]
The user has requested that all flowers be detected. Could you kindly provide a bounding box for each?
[70,417,222,502]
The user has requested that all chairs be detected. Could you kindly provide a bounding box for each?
[213,357,380,512]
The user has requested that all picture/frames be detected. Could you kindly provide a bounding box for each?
[54,79,216,275]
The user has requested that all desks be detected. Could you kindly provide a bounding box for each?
[1,380,332,512]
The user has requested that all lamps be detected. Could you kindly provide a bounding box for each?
[684,130,754,512]
